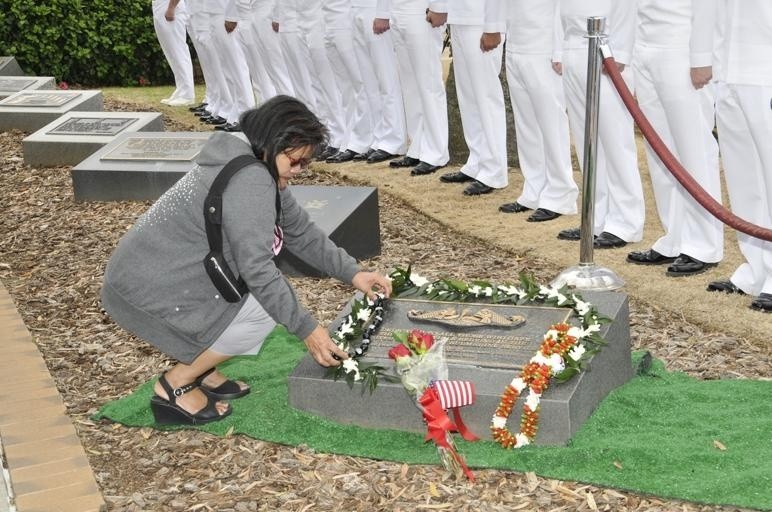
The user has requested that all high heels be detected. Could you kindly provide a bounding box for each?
[196,364,251,400]
[150,371,233,426]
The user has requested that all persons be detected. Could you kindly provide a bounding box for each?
[100,95,393,428]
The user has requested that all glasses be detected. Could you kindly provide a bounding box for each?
[282,150,311,168]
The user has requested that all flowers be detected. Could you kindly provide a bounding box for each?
[487,318,585,446]
[384,327,469,484]
[318,261,617,388]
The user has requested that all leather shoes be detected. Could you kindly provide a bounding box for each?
[187,103,242,131]
[315,145,439,175]
[707,281,747,297]
[593,231,628,249]
[463,180,494,195]
[558,225,582,242]
[439,172,473,181]
[665,252,718,277]
[499,202,529,213]
[160,94,195,106]
[749,293,771,312]
[527,208,559,221]
[626,247,679,265]
